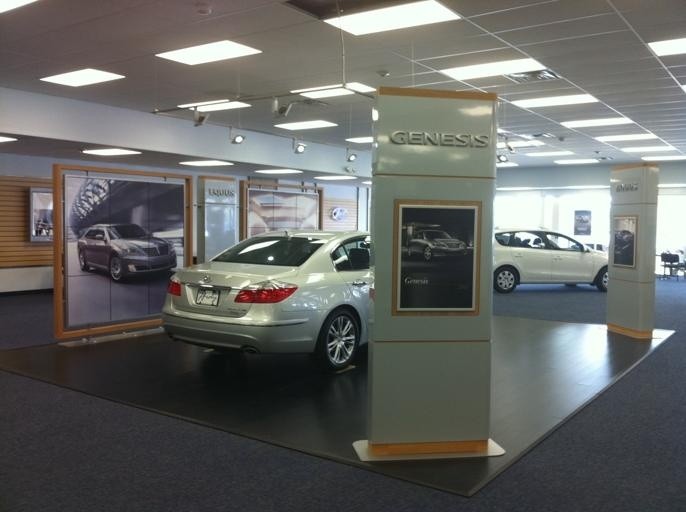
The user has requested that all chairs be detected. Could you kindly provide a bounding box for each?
[661,253,684,278]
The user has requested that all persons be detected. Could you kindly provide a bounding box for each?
[36,216,50,236]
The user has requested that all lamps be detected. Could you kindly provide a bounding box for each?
[272,99,292,119]
[228,125,245,144]
[497,131,513,163]
[345,101,358,163]
[193,107,210,126]
[292,131,306,155]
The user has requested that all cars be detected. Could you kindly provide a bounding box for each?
[617,230,634,245]
[492,227,609,294]
[408,229,469,262]
[577,215,590,223]
[77,224,177,281]
[159,230,376,369]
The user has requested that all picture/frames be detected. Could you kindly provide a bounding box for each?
[613,215,637,269]
[392,198,481,316]
